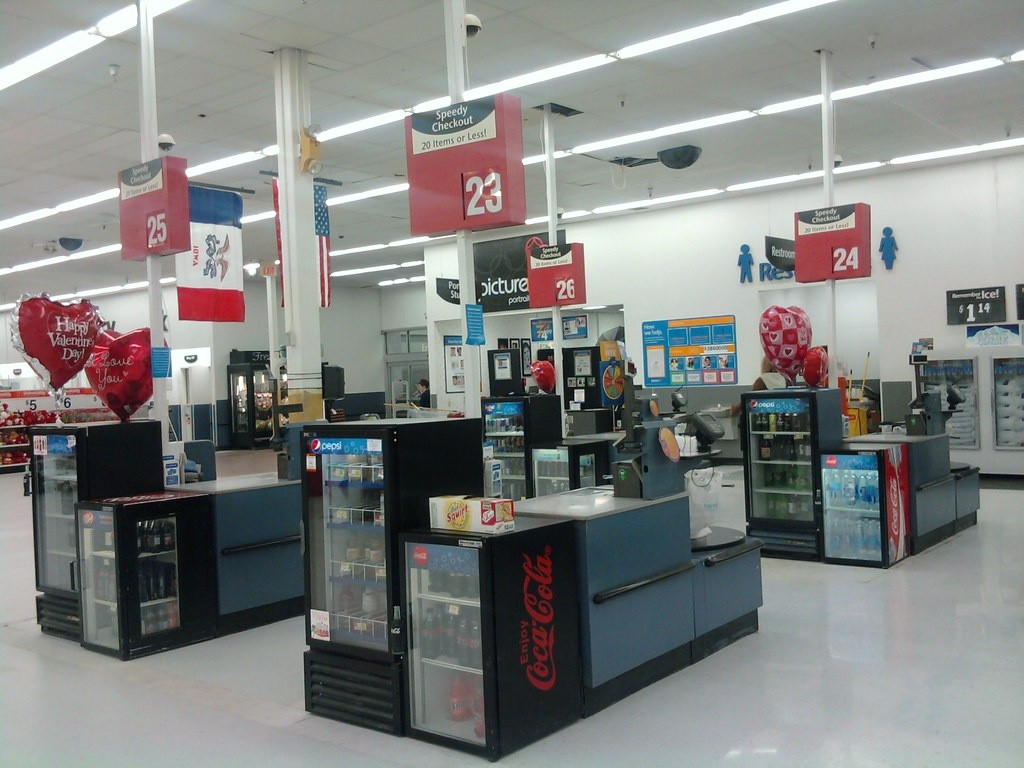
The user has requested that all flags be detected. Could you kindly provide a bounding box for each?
[270,179,332,309]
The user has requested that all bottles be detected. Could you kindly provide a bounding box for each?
[826,473,879,558]
[420,570,484,739]
[99,519,179,636]
[754,412,814,521]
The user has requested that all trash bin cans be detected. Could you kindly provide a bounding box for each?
[846,384,881,433]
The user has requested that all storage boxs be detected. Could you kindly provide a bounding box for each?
[428,493,514,533]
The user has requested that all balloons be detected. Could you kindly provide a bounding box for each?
[802,346,829,387]
[533,360,556,393]
[8,292,99,402]
[83,326,168,423]
[759,305,812,382]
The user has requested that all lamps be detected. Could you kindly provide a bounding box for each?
[301,124,324,175]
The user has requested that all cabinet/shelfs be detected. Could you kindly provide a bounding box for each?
[0,426,31,474]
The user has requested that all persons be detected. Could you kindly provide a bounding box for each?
[417,379,430,408]
[523,342,531,374]
[669,356,728,369]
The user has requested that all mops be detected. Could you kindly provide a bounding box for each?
[848,369,852,403]
[859,352,870,401]
